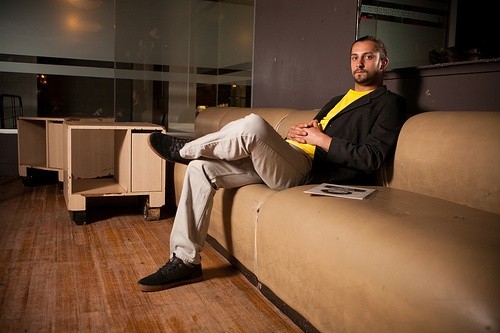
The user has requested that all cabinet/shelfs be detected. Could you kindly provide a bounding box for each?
[62,122,166,222]
[16,117,114,186]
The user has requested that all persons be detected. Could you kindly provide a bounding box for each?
[138,34,405,292]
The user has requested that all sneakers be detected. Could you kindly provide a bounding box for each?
[147,131,195,165]
[138,252,202,290]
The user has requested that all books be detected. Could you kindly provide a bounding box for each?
[303,181,378,201]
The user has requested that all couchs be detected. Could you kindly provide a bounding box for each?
[171,106,500,333]
